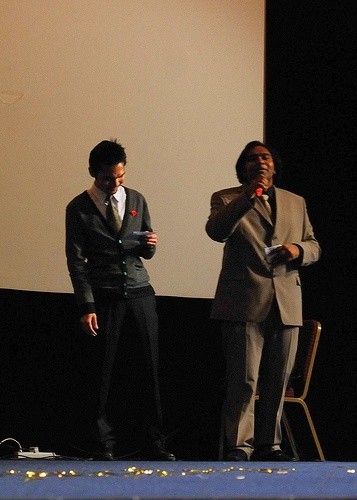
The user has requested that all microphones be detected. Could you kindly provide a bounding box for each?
[256,176,267,197]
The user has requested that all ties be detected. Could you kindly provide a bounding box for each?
[262,194,273,218]
[106,195,122,234]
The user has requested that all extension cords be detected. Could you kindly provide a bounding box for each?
[15,451,56,459]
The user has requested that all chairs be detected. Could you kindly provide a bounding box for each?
[218,320,326,462]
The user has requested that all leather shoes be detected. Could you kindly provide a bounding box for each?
[94,443,115,462]
[224,449,249,462]
[137,445,175,462]
[267,450,297,462]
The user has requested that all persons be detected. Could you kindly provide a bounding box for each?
[65,140,176,461]
[205,141,322,462]
